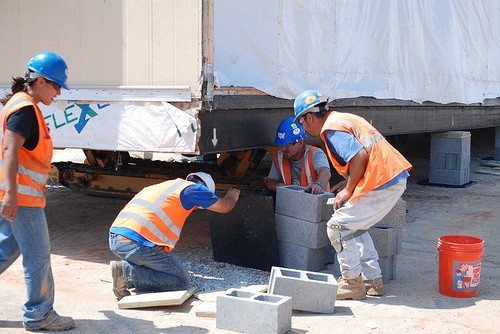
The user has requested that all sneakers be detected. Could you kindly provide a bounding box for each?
[363,276,384,296]
[334,272,366,300]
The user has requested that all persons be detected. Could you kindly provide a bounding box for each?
[266,118,332,195]
[0,51,75,332]
[109,172,241,301]
[292,90,414,300]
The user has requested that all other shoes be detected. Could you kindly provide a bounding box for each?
[109,260,131,299]
[24,308,75,331]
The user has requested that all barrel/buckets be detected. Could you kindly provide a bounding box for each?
[436,235,486,297]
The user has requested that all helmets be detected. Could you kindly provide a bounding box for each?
[185,171,215,210]
[292,89,328,123]
[274,116,308,145]
[24,51,69,90]
